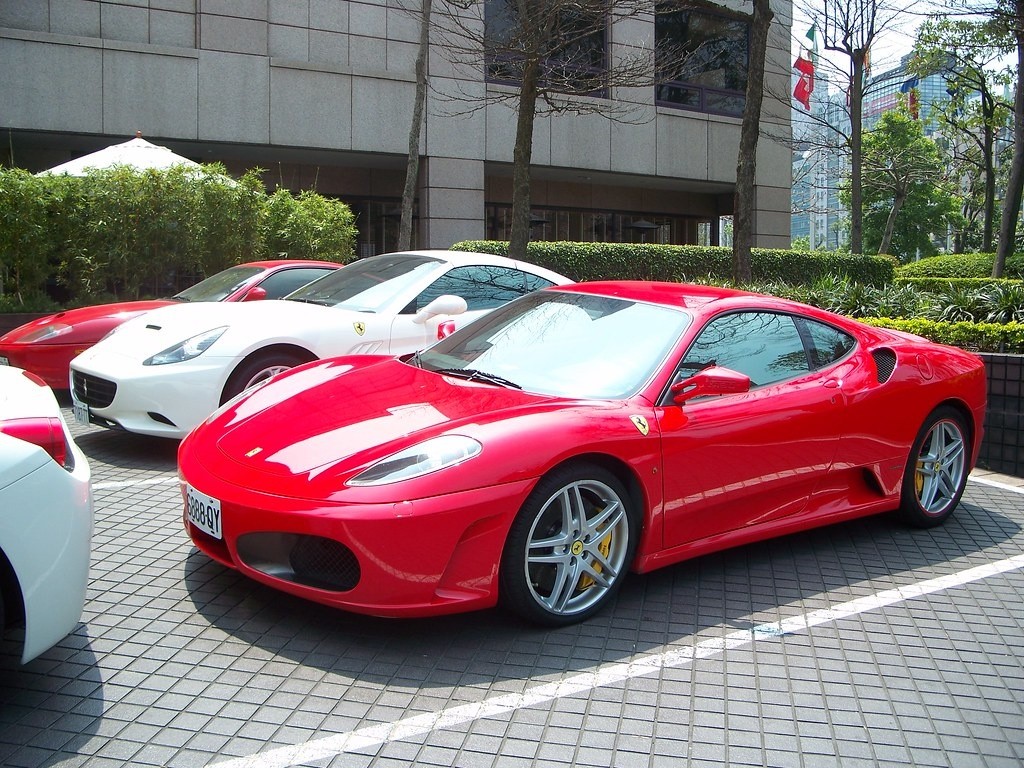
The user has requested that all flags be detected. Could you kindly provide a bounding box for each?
[900,52,920,119]
[793,24,819,111]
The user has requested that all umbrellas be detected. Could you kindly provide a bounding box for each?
[29,138,268,199]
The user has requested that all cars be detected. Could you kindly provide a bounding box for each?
[0,359,94,691]
[0,258,387,390]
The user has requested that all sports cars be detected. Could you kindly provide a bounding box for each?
[172,283,990,630]
[69,248,578,438]
